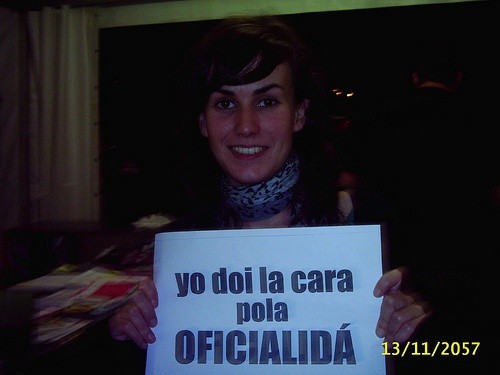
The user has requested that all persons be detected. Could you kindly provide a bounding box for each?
[334,166,378,226]
[22,14,444,375]
